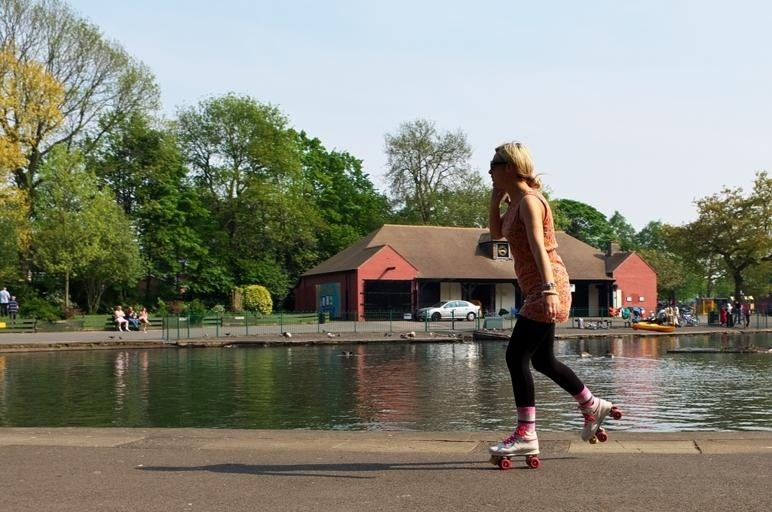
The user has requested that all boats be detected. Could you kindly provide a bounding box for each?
[633,320,675,333]
[667,346,759,353]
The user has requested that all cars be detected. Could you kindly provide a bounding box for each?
[416,299,483,323]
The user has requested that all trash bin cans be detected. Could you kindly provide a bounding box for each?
[708,312,720,326]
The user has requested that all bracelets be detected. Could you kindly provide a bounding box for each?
[542,282,556,291]
[541,291,559,296]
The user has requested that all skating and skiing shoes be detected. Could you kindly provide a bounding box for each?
[578,396,622,444]
[488,429,540,470]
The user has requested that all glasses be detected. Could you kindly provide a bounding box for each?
[490,161,508,170]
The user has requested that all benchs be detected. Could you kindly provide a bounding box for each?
[0,319,37,332]
[575,317,613,328]
[105,317,163,330]
[202,317,224,326]
[624,320,657,327]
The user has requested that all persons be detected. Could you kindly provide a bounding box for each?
[124,307,139,331]
[6,296,19,324]
[139,307,152,334]
[484,137,614,459]
[622,297,752,328]
[115,305,130,332]
[0,287,11,317]
